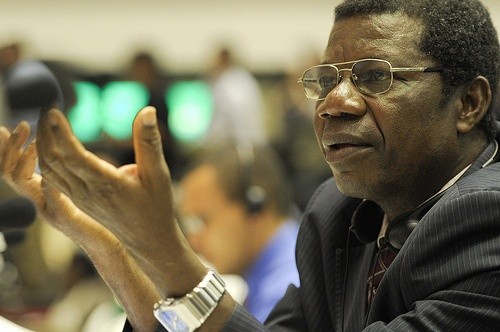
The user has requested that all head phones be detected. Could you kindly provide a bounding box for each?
[346,136,500,253]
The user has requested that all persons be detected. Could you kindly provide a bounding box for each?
[0,1,500,331]
[175,153,303,325]
[116,48,189,184]
[207,47,266,159]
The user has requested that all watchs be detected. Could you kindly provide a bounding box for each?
[152,266,227,331]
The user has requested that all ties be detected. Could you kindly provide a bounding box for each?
[369,250,395,304]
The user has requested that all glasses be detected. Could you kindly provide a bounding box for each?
[297,58,441,101]
